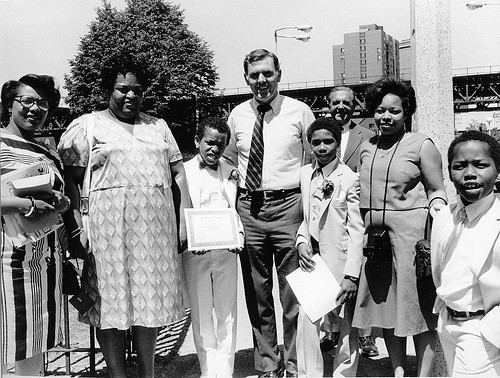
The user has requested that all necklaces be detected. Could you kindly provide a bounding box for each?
[377,136,400,158]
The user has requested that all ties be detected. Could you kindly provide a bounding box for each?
[245,105,272,192]
[336,145,342,160]
[439,209,467,272]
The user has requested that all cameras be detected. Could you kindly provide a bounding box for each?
[363,229,386,268]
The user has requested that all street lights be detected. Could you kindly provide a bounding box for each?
[274,25,313,58]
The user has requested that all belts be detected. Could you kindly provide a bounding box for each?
[446,306,485,318]
[237,187,299,195]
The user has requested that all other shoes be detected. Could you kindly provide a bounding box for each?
[286,370,298,378]
[358,336,379,356]
[258,365,283,378]
[320,330,340,352]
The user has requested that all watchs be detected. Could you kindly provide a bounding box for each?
[64,196,71,213]
[344,275,358,282]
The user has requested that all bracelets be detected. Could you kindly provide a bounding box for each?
[67,226,84,239]
[21,195,35,218]
[428,197,448,209]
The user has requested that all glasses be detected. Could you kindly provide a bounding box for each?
[16,95,50,111]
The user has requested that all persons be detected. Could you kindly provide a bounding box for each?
[183,117,244,378]
[218,50,447,378]
[0,73,72,378]
[56,55,191,378]
[429,131,500,378]
